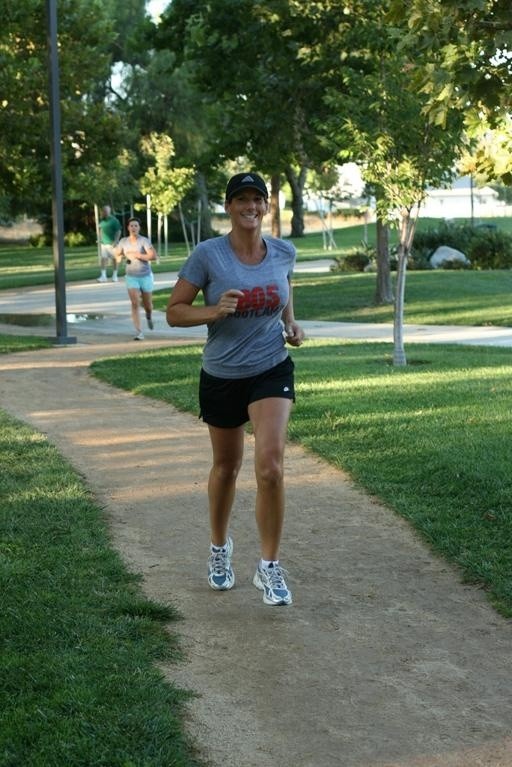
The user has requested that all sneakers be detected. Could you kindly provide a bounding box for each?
[252,559,293,606]
[132,331,144,340]
[207,536,235,591]
[145,314,154,330]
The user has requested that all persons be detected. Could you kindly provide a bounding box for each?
[166,173,305,606]
[114,218,157,340]
[96,205,122,282]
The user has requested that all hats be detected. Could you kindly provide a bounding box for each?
[226,173,269,200]
